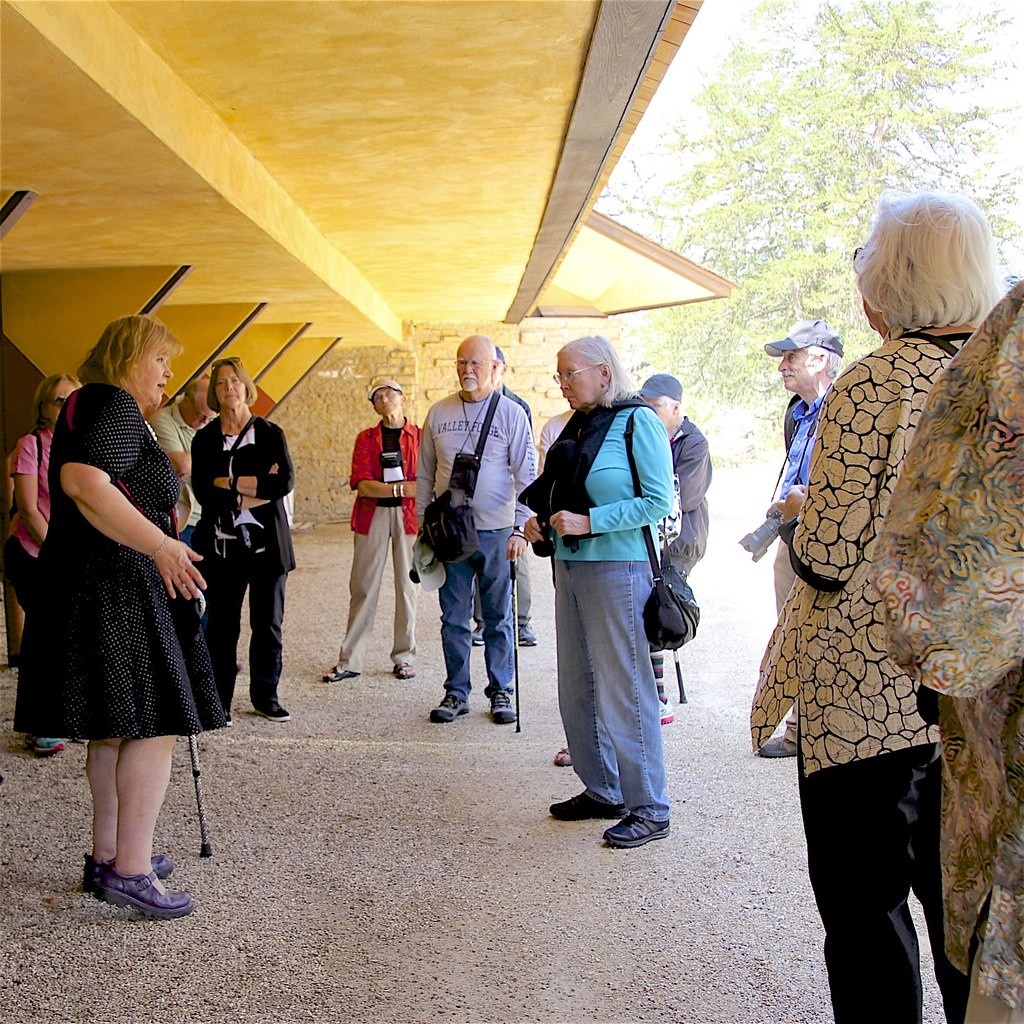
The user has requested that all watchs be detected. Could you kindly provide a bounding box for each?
[514,525,524,534]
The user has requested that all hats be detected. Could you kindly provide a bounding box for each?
[635,373,682,402]
[367,379,403,400]
[764,319,844,358]
[496,346,505,363]
[412,532,447,591]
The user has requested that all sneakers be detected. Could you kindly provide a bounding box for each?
[601,812,670,847]
[322,628,536,724]
[254,701,290,722]
[221,709,234,727]
[550,789,628,820]
[24,732,64,753]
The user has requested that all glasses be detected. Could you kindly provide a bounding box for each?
[186,394,214,425]
[453,358,496,369]
[851,247,866,276]
[48,396,66,408]
[553,364,604,384]
[211,356,241,372]
[374,391,401,401]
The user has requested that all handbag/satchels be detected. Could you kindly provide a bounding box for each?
[655,472,682,551]
[642,562,700,650]
[416,489,480,564]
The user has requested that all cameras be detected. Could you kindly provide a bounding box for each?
[737,508,784,562]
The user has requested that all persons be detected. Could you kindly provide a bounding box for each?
[0,372,81,755]
[322,380,423,683]
[753,319,844,757]
[15,316,228,920]
[749,188,1005,1024]
[189,354,298,729]
[149,377,216,547]
[469,347,537,647]
[418,333,538,724]
[869,276,1024,1023]
[522,334,712,849]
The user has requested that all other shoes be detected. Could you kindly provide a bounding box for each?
[658,701,672,725]
[554,743,572,767]
[83,850,174,894]
[92,864,194,917]
[757,736,799,757]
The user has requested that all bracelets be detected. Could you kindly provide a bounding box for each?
[228,476,239,490]
[393,483,405,498]
[239,494,243,507]
[151,535,167,559]
